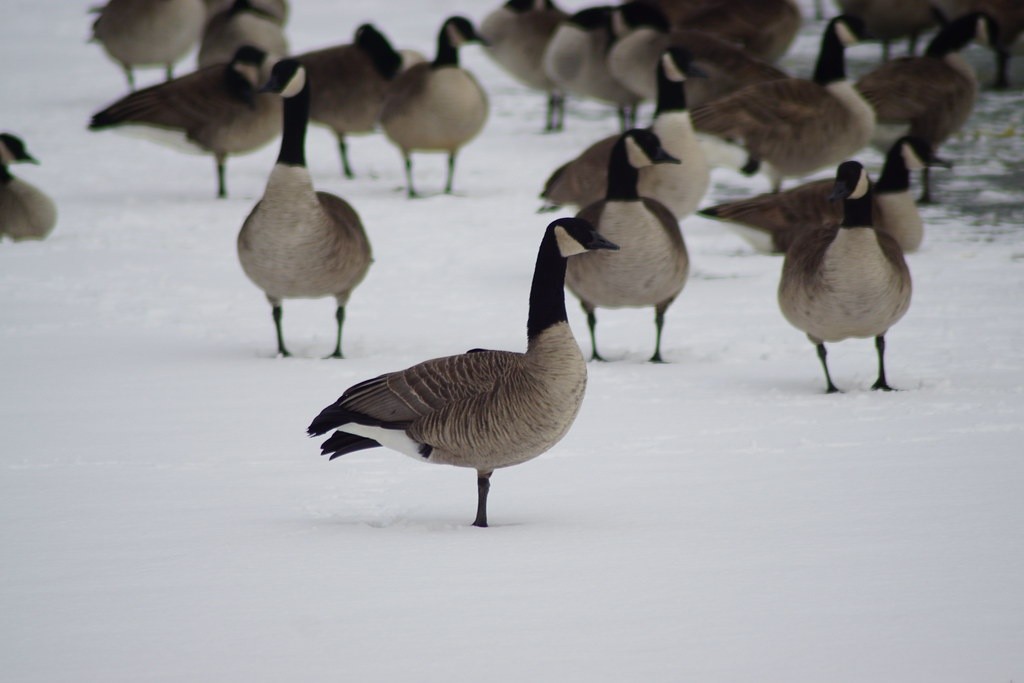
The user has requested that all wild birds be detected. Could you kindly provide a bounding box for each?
[277,22,425,177]
[533,47,712,224]
[779,160,913,396]
[557,128,689,363]
[481,0,1023,136]
[694,136,953,258]
[693,15,877,192]
[382,15,493,197]
[0,132,58,242]
[84,0,206,92]
[89,45,288,198]
[235,59,373,359]
[849,12,1012,207]
[202,0,289,68]
[307,217,621,532]
